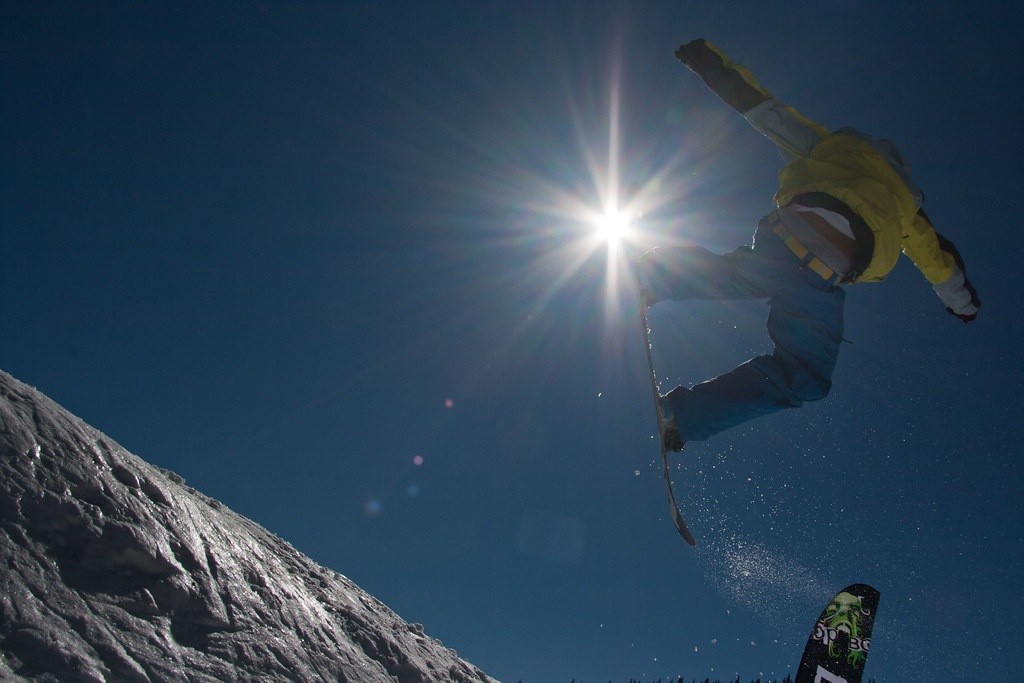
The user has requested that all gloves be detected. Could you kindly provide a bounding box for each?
[673,37,703,61]
[945,306,977,324]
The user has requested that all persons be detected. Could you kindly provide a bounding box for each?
[631,39,981,451]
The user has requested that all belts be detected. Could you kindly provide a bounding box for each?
[769,210,843,286]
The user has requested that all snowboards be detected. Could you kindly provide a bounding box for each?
[797,584,884,683]
[619,236,699,547]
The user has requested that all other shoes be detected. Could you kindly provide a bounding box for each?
[638,251,665,305]
[660,395,685,454]
